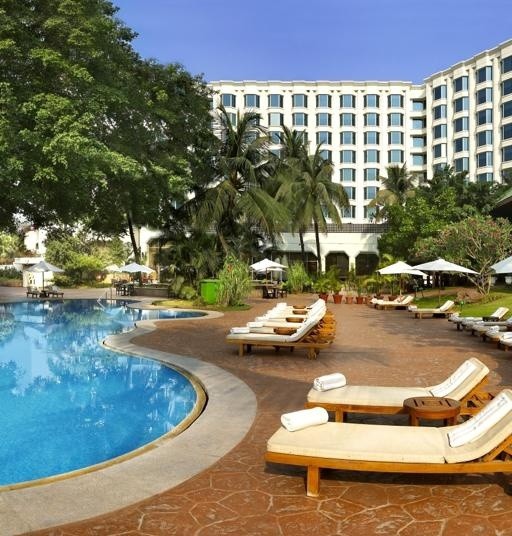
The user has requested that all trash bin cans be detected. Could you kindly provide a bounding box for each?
[199,280,218,304]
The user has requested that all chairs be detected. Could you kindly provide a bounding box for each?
[261,285,274,298]
[115,283,125,294]
[126,285,134,295]
[278,284,288,299]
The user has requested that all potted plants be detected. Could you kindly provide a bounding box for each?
[377,279,385,300]
[365,279,376,306]
[356,280,364,304]
[329,272,343,304]
[345,280,353,303]
[389,277,397,300]
[313,278,330,304]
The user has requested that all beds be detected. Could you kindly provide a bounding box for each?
[375,295,413,310]
[447,306,509,330]
[265,305,337,325]
[414,299,454,319]
[255,305,335,334]
[461,316,511,332]
[483,330,512,352]
[46,286,66,298]
[26,286,42,298]
[273,298,336,317]
[265,390,511,499]
[228,311,335,359]
[371,295,404,308]
[303,357,496,422]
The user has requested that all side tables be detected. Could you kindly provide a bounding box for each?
[402,396,461,426]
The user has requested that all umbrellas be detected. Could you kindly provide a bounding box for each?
[25,261,64,291]
[250,259,288,286]
[120,263,152,284]
[379,261,428,299]
[407,259,480,307]
